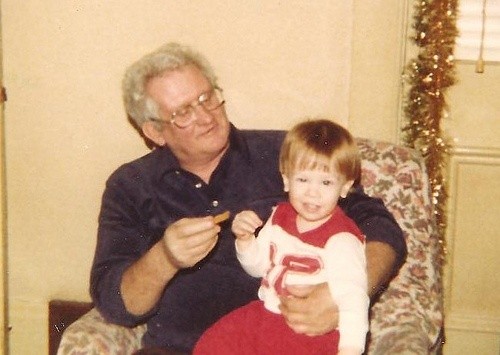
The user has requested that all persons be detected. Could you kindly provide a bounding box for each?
[192,119,370,355]
[89,43,408,355]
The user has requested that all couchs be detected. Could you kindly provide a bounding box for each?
[55,138,445,355]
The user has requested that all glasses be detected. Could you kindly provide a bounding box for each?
[148,86,226,132]
